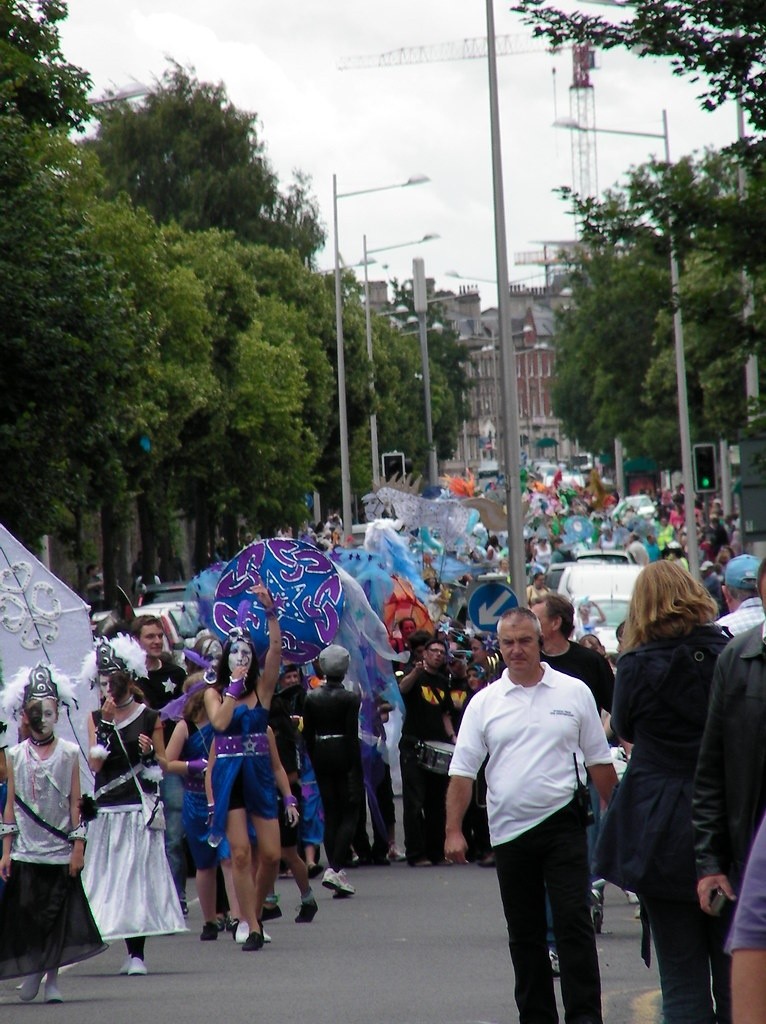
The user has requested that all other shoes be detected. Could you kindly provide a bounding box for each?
[120,954,147,975]
[200,921,218,940]
[260,906,281,920]
[235,923,271,944]
[295,899,317,923]
[19,971,45,1001]
[308,864,323,878]
[43,983,62,1003]
[242,929,265,950]
[274,854,451,877]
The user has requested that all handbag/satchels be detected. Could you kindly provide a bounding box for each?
[141,791,166,831]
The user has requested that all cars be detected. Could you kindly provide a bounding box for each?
[543,549,647,660]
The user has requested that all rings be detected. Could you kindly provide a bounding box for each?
[259,584,260,587]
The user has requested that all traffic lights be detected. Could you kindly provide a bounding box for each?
[694,442,718,494]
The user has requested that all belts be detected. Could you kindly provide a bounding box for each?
[318,734,344,740]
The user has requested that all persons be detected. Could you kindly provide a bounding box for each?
[1,466,766,1024]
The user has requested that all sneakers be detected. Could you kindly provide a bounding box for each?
[322,868,355,894]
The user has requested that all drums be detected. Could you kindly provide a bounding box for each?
[416,740,458,775]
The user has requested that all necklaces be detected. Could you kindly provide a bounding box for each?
[30,733,55,746]
[114,694,134,710]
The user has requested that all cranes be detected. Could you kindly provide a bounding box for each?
[350,27,602,233]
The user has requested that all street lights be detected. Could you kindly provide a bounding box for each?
[332,172,433,547]
[458,324,534,476]
[550,115,701,579]
[362,231,442,492]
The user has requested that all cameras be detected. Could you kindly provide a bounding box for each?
[709,887,727,914]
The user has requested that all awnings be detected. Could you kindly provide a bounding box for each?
[535,436,559,447]
[599,454,657,471]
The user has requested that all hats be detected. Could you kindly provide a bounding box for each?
[319,644,351,676]
[667,540,682,549]
[183,634,223,667]
[700,560,713,570]
[726,554,761,589]
[80,635,148,678]
[0,661,79,720]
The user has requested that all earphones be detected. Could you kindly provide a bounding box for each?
[538,638,544,646]
